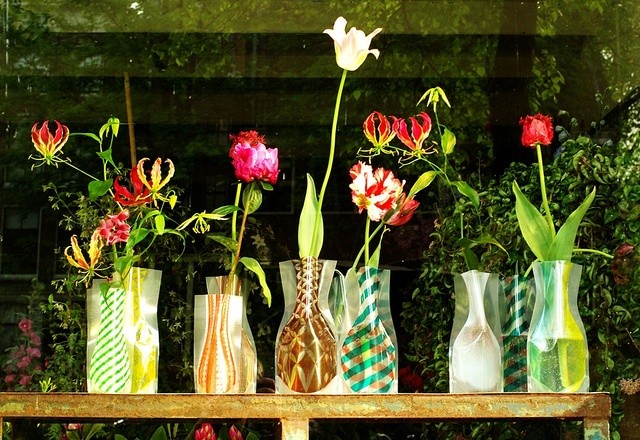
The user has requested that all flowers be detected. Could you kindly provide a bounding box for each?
[355,85,510,272]
[205,142,281,307]
[296,15,385,259]
[511,111,617,263]
[28,111,229,282]
[348,159,407,269]
[225,127,267,274]
[350,169,443,269]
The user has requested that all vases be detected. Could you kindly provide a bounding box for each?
[526,259,591,393]
[84,288,134,395]
[273,259,343,395]
[204,274,258,394]
[338,266,400,394]
[92,265,164,395]
[447,268,505,393]
[193,293,244,394]
